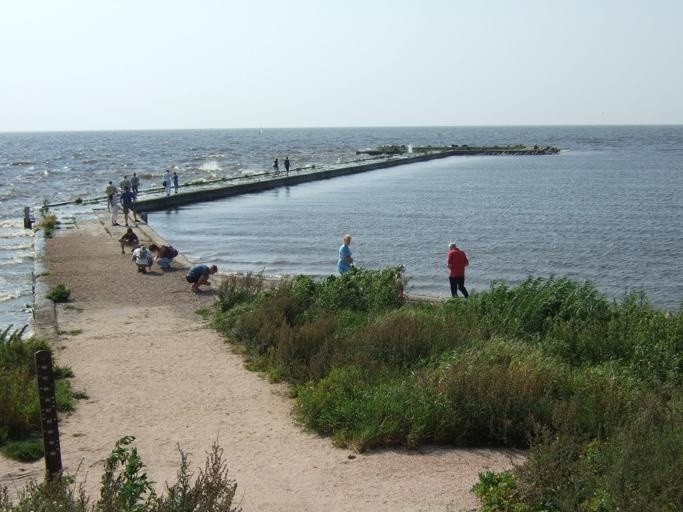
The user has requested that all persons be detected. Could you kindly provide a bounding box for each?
[173,207,178,214]
[336,233,354,276]
[285,186,289,195]
[445,242,469,298]
[273,188,278,195]
[272,158,280,175]
[117,227,139,256]
[121,175,131,192]
[105,180,116,213]
[162,169,172,196]
[109,185,120,226]
[129,171,140,194]
[165,209,172,215]
[131,243,153,272]
[283,156,290,176]
[119,185,140,227]
[171,171,179,193]
[184,263,217,294]
[148,243,172,270]
